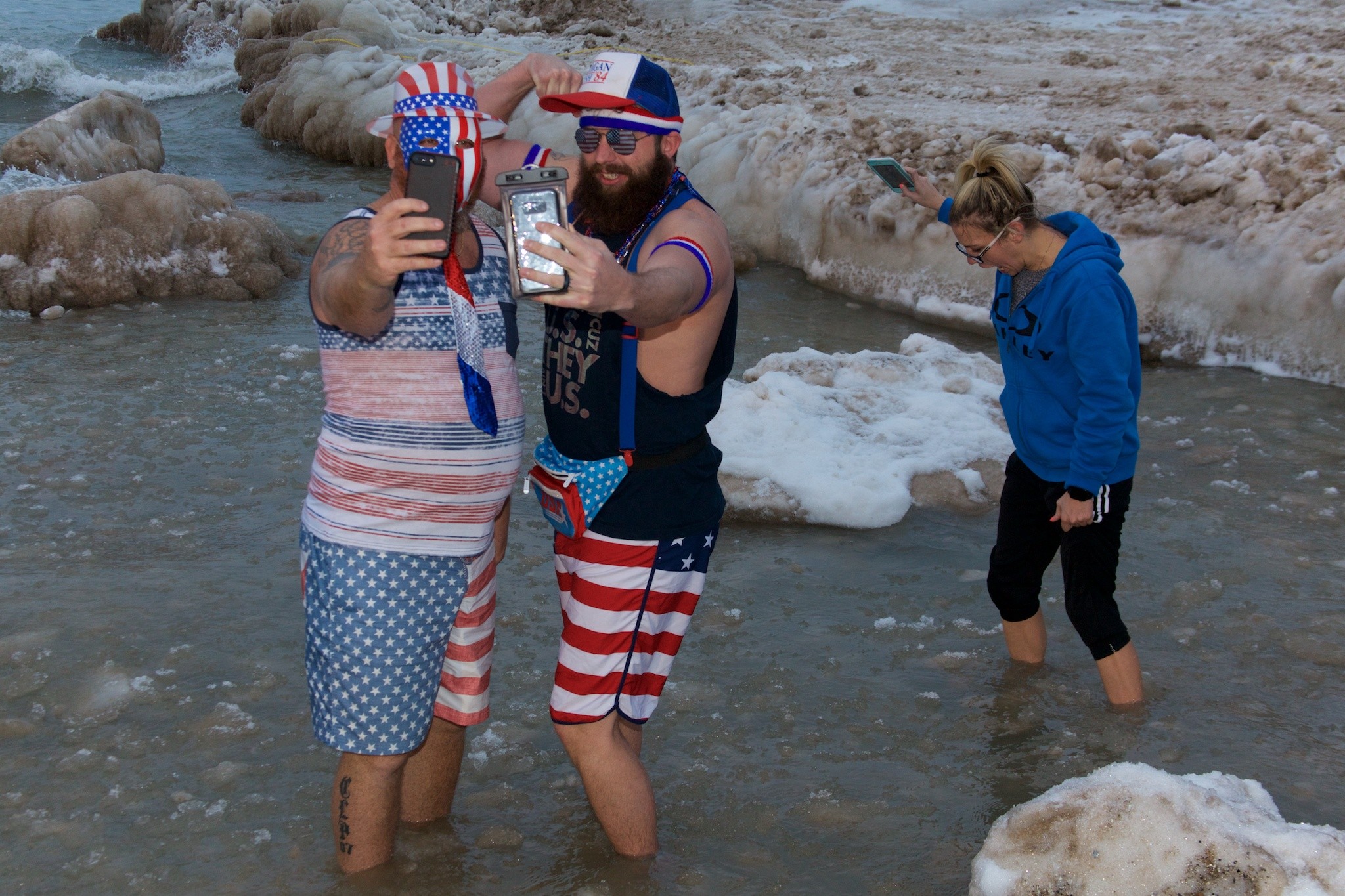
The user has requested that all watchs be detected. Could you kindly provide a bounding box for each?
[1067,487,1093,501]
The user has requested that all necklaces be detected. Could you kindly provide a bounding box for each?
[586,170,681,262]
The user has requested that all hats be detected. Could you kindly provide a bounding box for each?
[538,52,681,119]
[364,62,509,140]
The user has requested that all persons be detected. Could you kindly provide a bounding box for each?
[297,61,525,874]
[899,134,1143,705]
[475,52,738,856]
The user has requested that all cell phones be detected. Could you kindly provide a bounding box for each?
[403,152,461,258]
[866,157,915,193]
[501,180,568,298]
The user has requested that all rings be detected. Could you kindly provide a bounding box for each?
[562,274,570,289]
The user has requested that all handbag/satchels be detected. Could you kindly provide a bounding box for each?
[523,435,706,539]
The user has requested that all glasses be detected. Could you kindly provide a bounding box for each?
[574,128,650,156]
[955,217,1021,264]
